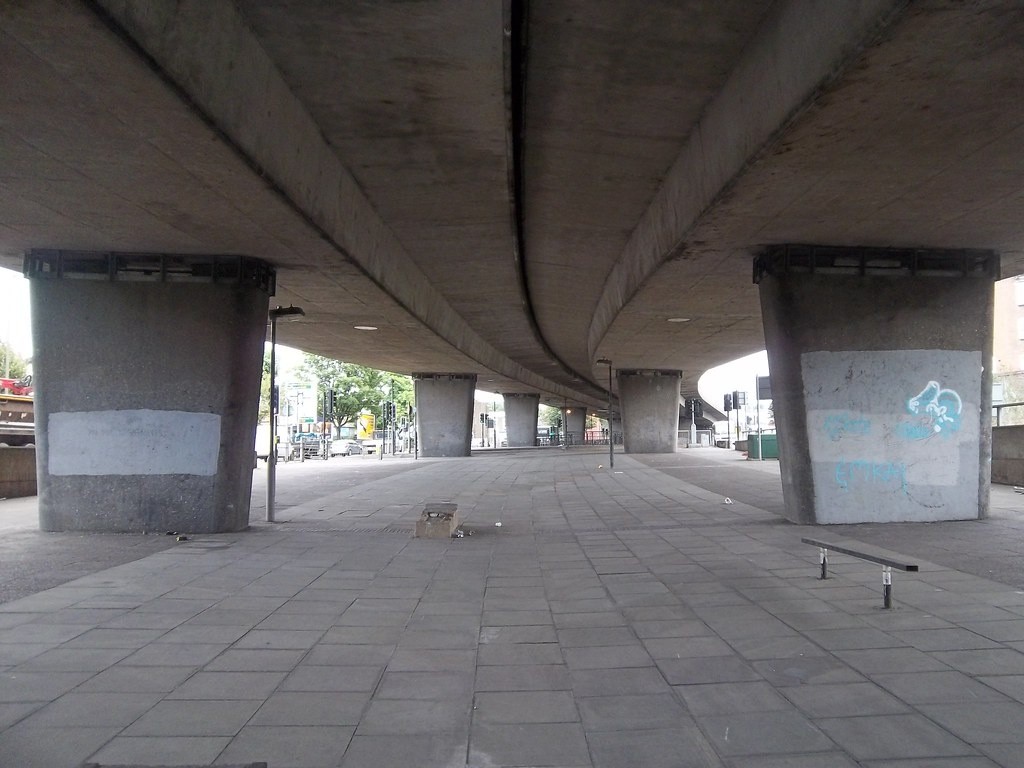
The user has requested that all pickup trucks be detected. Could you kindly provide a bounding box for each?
[362,430,405,454]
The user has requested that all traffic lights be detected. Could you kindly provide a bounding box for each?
[557,419,562,427]
[480,413,484,423]
[389,402,392,415]
[268,387,276,407]
[333,392,336,406]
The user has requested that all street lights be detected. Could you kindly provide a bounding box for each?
[596,359,614,468]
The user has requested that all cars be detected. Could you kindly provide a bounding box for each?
[330,439,362,457]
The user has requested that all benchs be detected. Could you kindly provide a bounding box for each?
[800,535,919,609]
